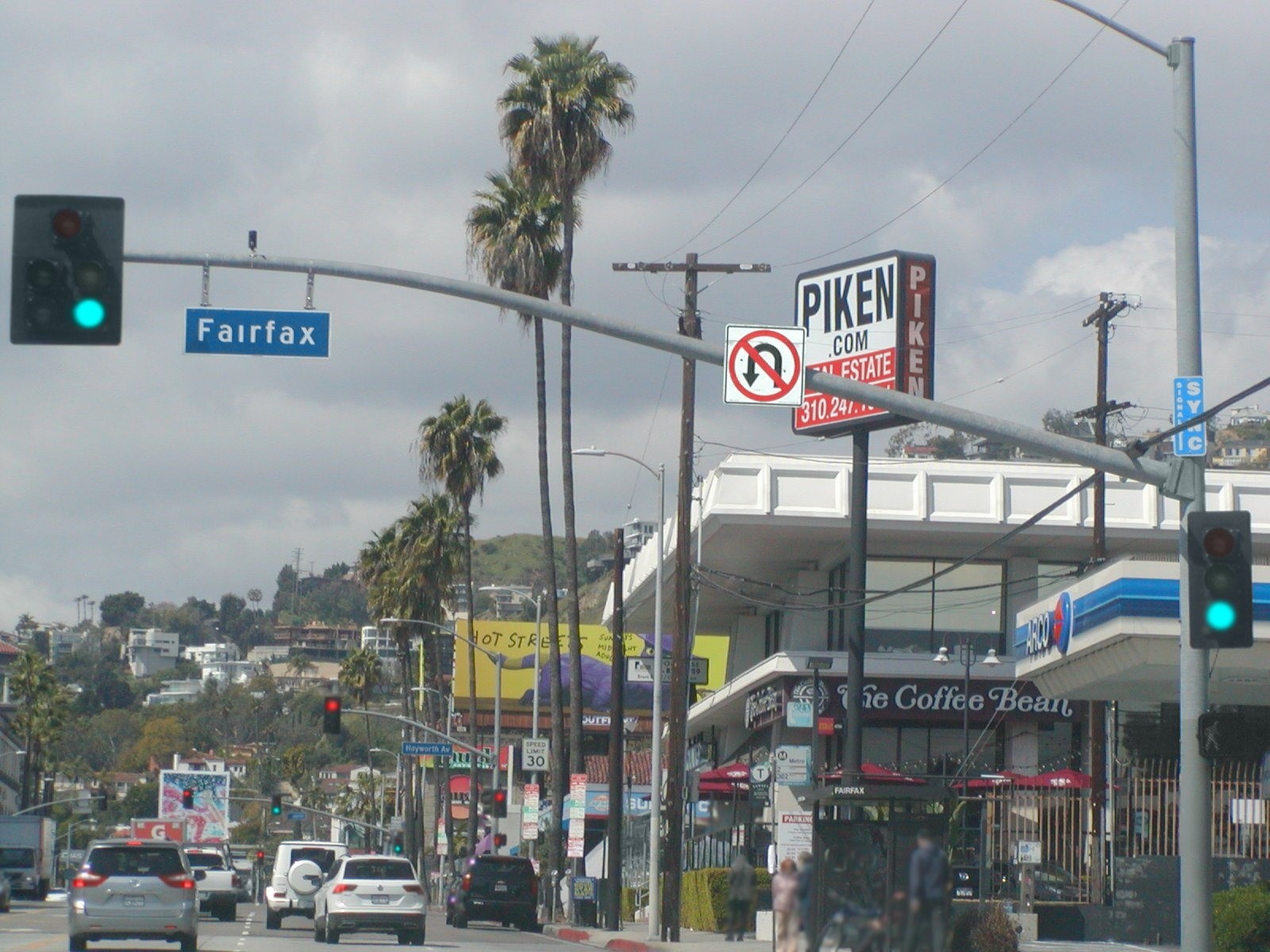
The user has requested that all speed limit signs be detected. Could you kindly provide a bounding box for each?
[521,738,551,772]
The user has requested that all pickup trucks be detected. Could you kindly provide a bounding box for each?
[180,843,238,922]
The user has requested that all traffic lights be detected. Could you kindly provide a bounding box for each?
[323,696,341,734]
[1197,710,1248,758]
[271,792,282,815]
[257,851,264,865]
[494,833,506,846]
[392,832,404,856]
[493,789,508,818]
[10,195,123,347]
[1187,509,1254,650]
[182,788,194,809]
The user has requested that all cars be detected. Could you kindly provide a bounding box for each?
[0,867,13,911]
[990,861,1091,904]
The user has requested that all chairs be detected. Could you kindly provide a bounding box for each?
[210,855,222,865]
[510,867,521,873]
[188,854,197,866]
[21,850,33,864]
[90,851,120,873]
[480,864,493,872]
[326,851,334,863]
[148,853,184,875]
[358,868,371,878]
[385,868,401,877]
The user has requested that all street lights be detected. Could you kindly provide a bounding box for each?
[569,443,665,941]
[933,630,1001,858]
[370,748,401,815]
[65,818,98,891]
[478,585,543,859]
[380,615,506,856]
[804,655,833,856]
[411,687,453,742]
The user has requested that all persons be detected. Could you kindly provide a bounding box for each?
[725,854,754,941]
[771,852,816,952]
[894,829,955,952]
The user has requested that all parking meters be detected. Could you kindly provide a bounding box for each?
[550,871,560,923]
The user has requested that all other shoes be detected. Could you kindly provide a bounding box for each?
[737,935,743,941]
[725,936,734,941]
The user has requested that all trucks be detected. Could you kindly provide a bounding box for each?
[0,814,57,901]
[131,819,187,872]
[232,859,254,902]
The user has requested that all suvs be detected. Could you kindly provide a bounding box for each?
[264,841,427,946]
[66,838,202,952]
[445,854,538,932]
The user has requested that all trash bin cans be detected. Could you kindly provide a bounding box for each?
[575,900,597,924]
